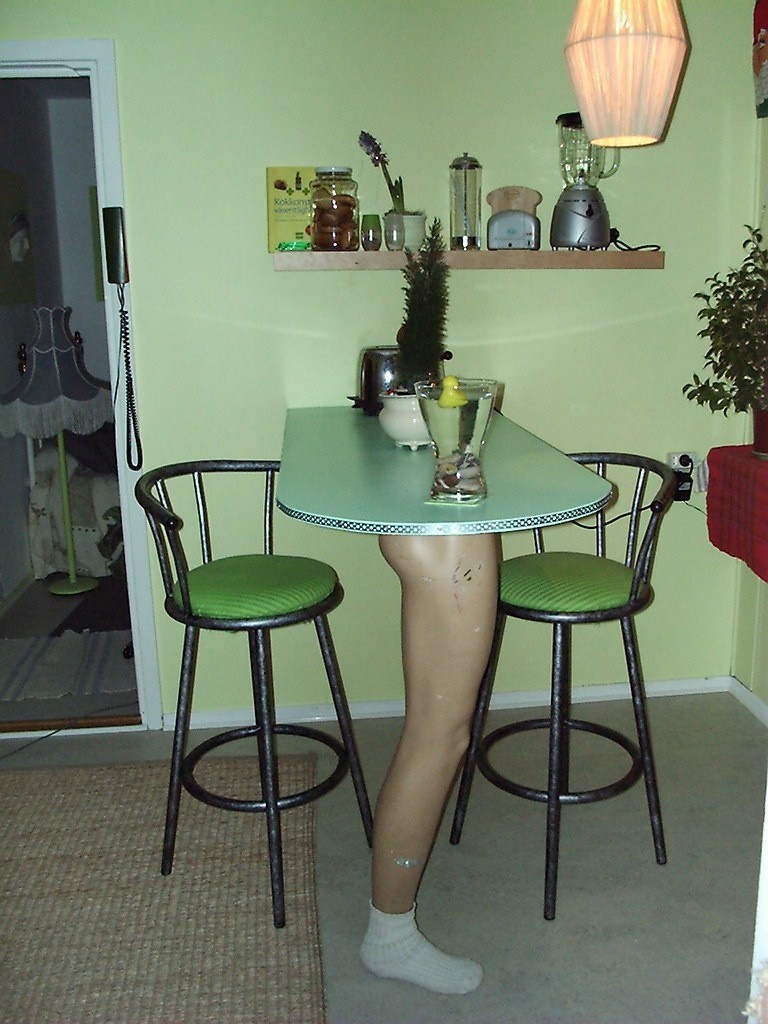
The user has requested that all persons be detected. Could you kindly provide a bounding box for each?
[361,532,499,994]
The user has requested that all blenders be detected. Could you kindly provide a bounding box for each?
[549,110,611,251]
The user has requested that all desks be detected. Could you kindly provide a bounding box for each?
[277,407,618,993]
[707,442,768,585]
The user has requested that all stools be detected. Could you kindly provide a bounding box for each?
[134,459,374,930]
[450,452,675,921]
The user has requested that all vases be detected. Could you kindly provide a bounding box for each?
[383,214,428,252]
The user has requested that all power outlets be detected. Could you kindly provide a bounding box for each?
[666,451,700,470]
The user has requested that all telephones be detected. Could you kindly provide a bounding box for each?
[103,206,130,285]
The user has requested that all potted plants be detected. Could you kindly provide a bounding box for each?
[376,216,455,450]
[682,223,768,451]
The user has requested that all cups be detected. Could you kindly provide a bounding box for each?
[361,214,381,251]
[413,377,506,506]
[448,152,482,250]
[384,213,406,250]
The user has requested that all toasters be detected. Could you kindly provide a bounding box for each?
[486,186,543,251]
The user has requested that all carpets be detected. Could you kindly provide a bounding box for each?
[0,629,136,701]
[0,751,329,1024]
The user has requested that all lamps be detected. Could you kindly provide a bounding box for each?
[1,306,114,596]
[563,0,689,147]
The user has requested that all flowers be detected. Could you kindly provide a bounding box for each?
[357,131,426,215]
